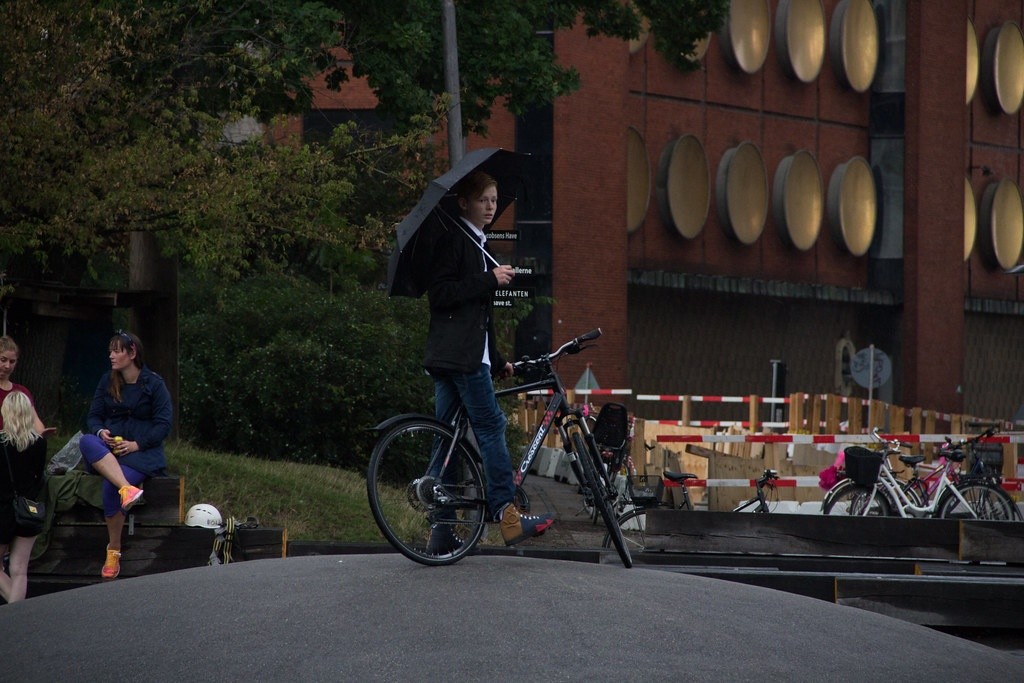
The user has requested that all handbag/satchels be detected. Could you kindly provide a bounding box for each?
[11,497,47,533]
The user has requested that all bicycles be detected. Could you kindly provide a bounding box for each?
[573,400,637,525]
[602,468,782,551]
[363,329,634,568]
[818,421,1024,521]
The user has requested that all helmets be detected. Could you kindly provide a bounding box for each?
[184,502,220,528]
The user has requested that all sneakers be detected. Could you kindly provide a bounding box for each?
[498,503,555,545]
[120,484,143,511]
[425,525,464,556]
[102,543,120,581]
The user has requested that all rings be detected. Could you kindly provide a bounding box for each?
[125,447,128,451]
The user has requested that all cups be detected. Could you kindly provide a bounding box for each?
[113,436,123,454]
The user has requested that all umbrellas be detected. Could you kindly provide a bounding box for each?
[380,141,533,301]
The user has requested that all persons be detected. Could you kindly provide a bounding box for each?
[0,334,58,442]
[421,174,558,559]
[0,390,49,604]
[79,327,174,581]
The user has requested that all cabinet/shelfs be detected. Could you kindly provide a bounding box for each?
[49,473,184,527]
